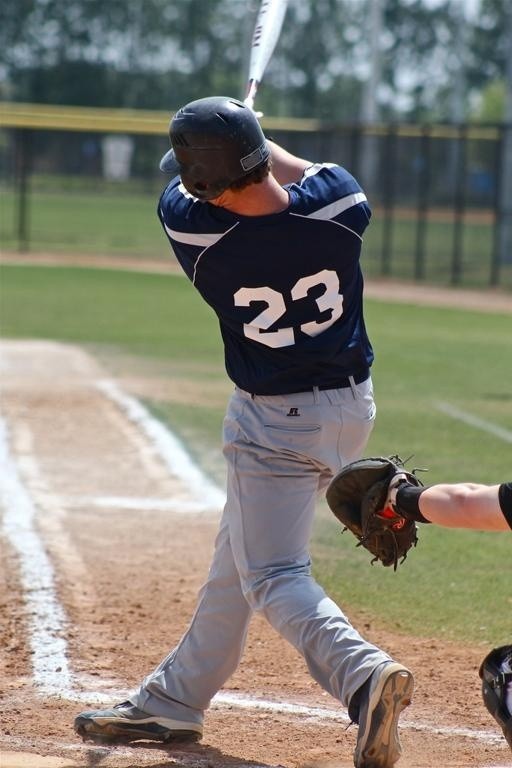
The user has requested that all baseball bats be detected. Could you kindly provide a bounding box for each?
[244,0,286,110]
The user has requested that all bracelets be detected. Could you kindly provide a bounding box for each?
[399,484,432,524]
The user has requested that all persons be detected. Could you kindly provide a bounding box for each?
[74,94,415,768]
[363,467,512,755]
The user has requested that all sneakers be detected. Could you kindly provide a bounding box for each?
[353,660,414,768]
[74,701,202,744]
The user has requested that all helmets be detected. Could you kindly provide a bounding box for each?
[159,96,271,200]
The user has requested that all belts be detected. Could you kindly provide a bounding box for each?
[290,368,372,391]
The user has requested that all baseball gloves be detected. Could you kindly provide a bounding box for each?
[326,455,418,568]
